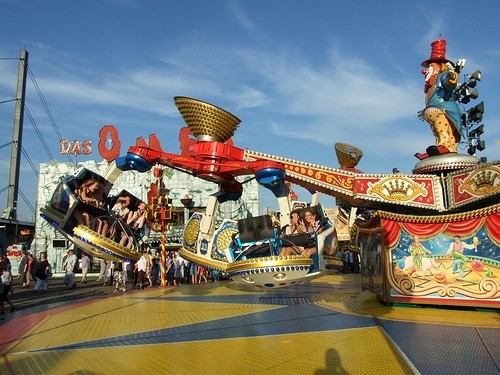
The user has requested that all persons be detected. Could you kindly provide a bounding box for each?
[63,250,77,289]
[97,251,230,294]
[80,252,90,284]
[18,250,49,294]
[336,247,360,275]
[279,209,323,258]
[73,180,147,249]
[0,253,15,315]
[415,38,462,160]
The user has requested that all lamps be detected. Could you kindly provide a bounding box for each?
[453,70,486,155]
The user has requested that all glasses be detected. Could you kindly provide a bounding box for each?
[290,216,293,219]
